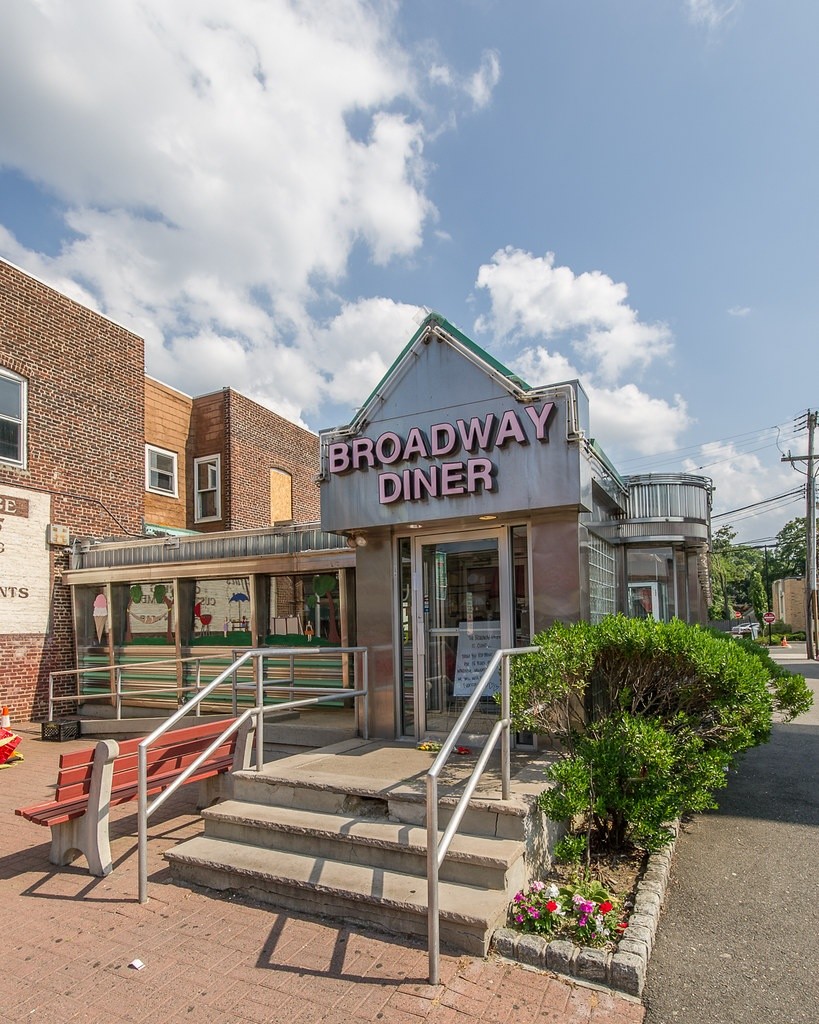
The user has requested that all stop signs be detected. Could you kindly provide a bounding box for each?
[763,612,775,623]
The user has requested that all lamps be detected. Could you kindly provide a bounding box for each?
[346,530,368,548]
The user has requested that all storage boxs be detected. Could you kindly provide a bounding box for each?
[41,719,81,743]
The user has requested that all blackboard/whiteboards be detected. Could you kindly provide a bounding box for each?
[453,621,501,697]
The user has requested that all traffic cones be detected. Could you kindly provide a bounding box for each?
[2,707,11,732]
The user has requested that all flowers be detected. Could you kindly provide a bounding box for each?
[416,736,470,755]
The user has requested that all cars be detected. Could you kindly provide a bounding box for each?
[732,622,760,634]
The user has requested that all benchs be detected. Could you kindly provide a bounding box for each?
[15,717,256,877]
